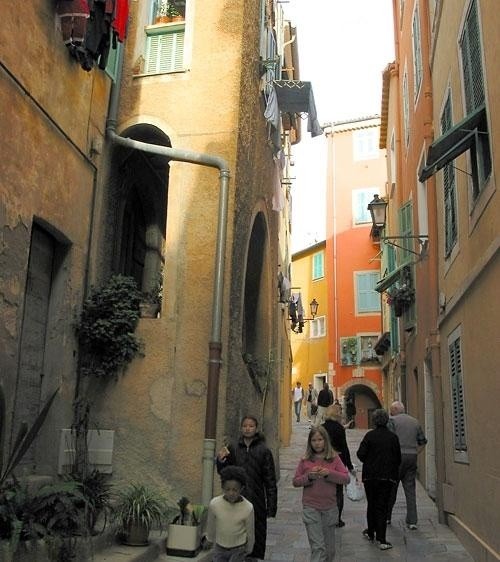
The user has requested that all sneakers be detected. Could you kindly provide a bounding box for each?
[297,416,314,429]
[363,519,417,551]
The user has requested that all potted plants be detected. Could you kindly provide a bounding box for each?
[133,285,159,319]
[111,480,165,549]
[67,467,114,539]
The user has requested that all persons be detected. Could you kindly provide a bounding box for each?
[305,383,319,422]
[291,381,305,422]
[385,399,428,530]
[312,382,334,427]
[214,416,278,561]
[355,407,402,550]
[321,405,359,528]
[203,464,257,561]
[292,423,352,561]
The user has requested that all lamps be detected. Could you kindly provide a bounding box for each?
[367,191,430,260]
[285,298,320,323]
